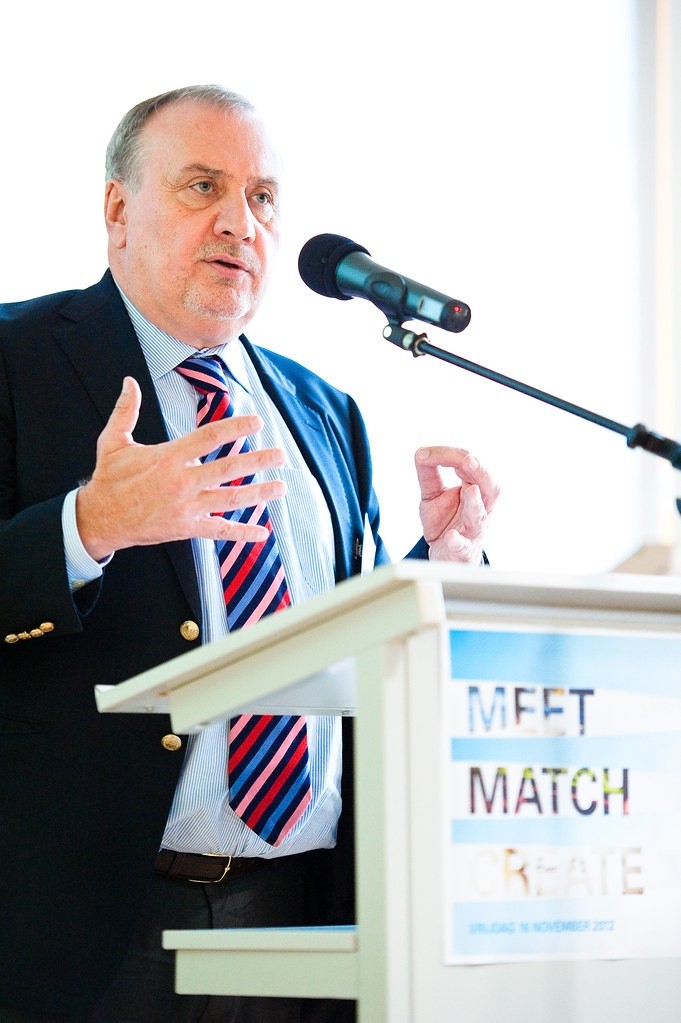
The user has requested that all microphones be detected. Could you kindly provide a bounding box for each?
[297,232,472,333]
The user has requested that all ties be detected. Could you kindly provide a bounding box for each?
[175,353,313,848]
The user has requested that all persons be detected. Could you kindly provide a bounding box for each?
[0,88,502,1023]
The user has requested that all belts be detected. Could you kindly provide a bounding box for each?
[158,849,334,884]
